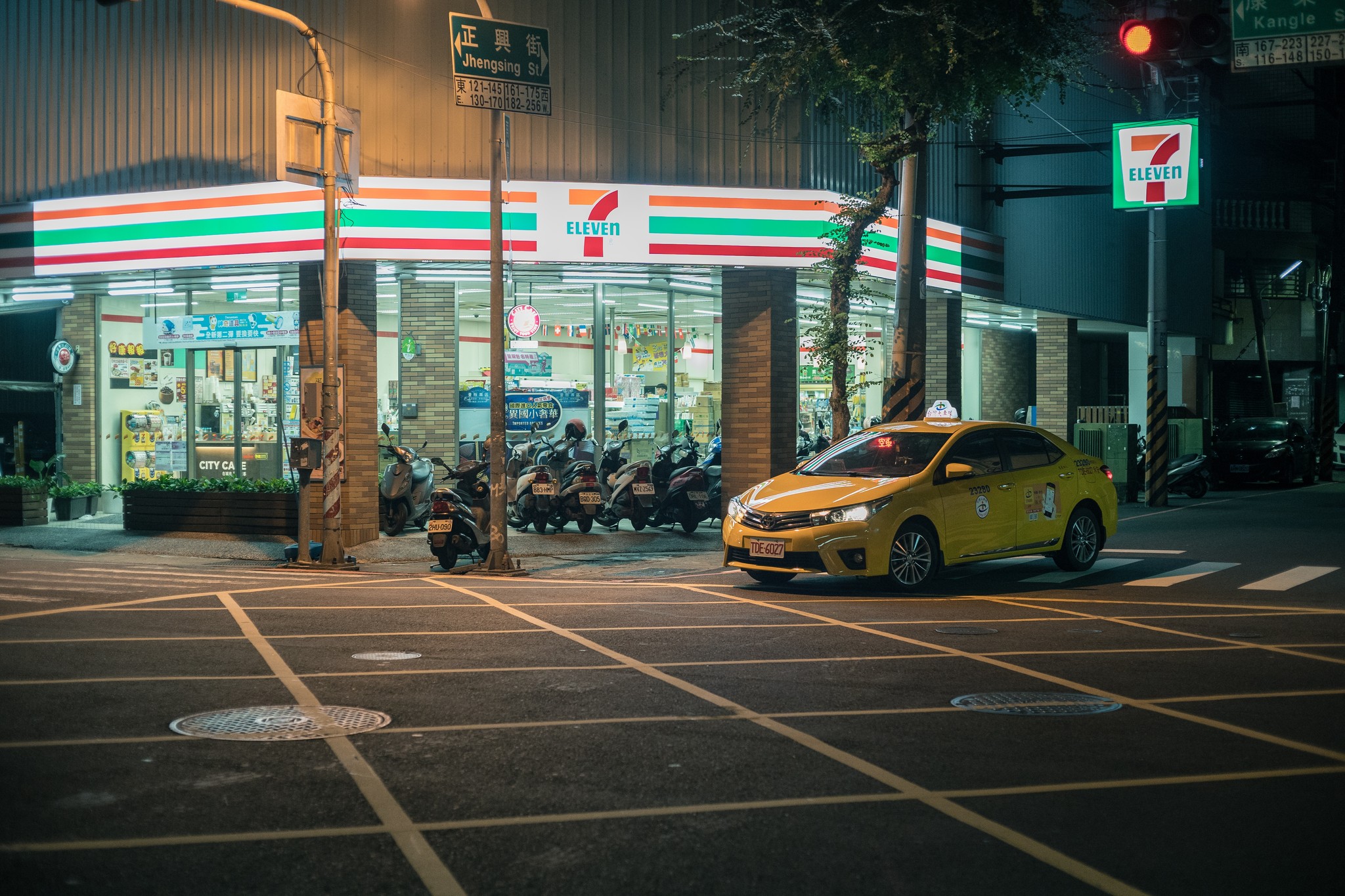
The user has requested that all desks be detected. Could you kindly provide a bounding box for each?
[181,441,278,481]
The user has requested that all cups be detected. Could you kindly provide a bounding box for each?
[242,354,251,371]
[250,408,255,425]
[209,359,220,378]
[244,382,256,403]
[163,352,171,366]
[205,375,222,403]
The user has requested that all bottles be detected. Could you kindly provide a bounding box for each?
[201,428,212,432]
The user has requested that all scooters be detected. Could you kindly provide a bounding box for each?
[427,438,505,572]
[482,417,832,537]
[378,423,435,537]
[1135,424,1209,499]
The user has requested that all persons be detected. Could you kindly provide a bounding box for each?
[656,383,667,396]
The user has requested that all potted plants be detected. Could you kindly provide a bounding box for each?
[0,452,107,527]
[105,473,299,535]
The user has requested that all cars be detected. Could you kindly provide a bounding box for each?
[1210,418,1316,487]
[723,400,1119,592]
[1332,422,1345,466]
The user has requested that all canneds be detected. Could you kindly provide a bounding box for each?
[803,370,808,380]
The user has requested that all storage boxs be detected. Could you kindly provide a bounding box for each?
[591,372,722,464]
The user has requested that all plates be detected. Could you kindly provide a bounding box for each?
[304,422,323,436]
[339,442,344,463]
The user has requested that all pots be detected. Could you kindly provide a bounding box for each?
[159,391,174,404]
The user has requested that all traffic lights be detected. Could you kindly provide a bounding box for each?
[1117,15,1232,69]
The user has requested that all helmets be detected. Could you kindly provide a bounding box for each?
[863,416,881,428]
[1015,410,1026,421]
[565,418,586,439]
[606,473,617,488]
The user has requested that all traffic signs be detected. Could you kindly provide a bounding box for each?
[450,12,552,116]
[1229,0,1345,74]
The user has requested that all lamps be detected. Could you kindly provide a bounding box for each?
[618,287,627,354]
[683,294,692,359]
